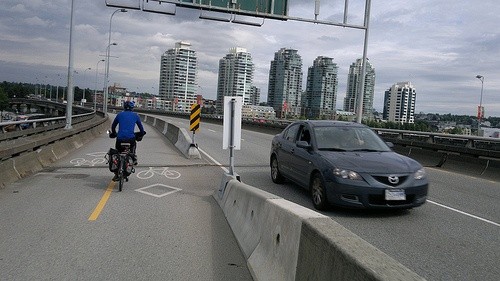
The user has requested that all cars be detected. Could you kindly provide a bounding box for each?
[269,120,430,214]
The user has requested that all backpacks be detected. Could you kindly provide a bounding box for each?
[121,155,133,176]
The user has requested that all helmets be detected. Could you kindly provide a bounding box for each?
[124,101,134,110]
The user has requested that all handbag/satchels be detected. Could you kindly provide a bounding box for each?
[104,148,121,172]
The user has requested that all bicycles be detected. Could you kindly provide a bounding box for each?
[106,130,141,192]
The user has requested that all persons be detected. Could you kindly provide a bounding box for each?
[109,101,144,166]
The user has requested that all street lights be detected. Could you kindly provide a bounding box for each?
[34,59,105,109]
[103,8,129,113]
[476,74,484,130]
